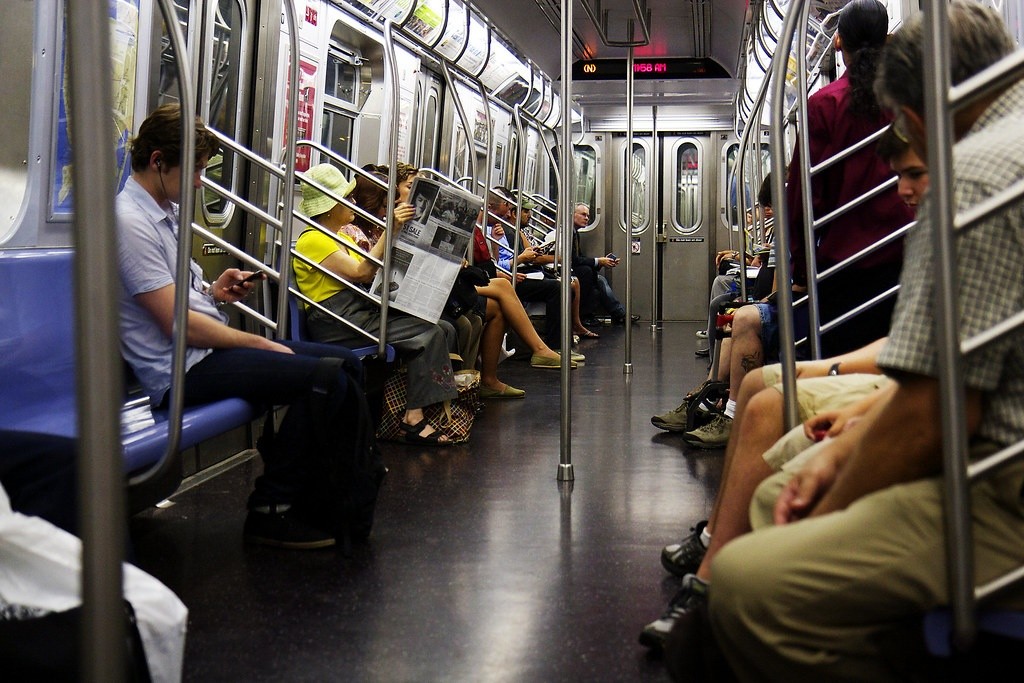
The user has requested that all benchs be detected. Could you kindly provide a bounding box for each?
[0,249,270,522]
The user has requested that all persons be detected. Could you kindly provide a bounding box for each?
[637,0,1024,683]
[472,186,641,344]
[338,172,487,411]
[411,187,476,255]
[113,103,368,550]
[379,161,577,398]
[293,163,464,445]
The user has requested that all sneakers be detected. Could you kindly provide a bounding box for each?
[660,520,710,577]
[681,413,734,449]
[640,572,710,646]
[650,401,713,431]
[242,511,335,549]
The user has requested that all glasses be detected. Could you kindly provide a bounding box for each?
[891,113,907,143]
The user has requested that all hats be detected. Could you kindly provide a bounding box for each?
[298,163,357,218]
[514,194,538,210]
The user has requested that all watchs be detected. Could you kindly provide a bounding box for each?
[733,251,737,255]
[827,363,841,376]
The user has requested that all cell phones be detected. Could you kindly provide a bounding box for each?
[606,253,616,261]
[229,270,264,292]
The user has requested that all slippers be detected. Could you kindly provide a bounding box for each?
[572,331,601,339]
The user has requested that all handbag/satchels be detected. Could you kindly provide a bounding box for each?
[376,369,482,444]
[255,372,386,543]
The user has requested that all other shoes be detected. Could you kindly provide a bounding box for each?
[478,381,526,398]
[612,315,641,324]
[530,355,578,369]
[580,317,601,326]
[552,348,586,361]
[694,348,709,355]
[682,386,703,400]
[695,330,708,338]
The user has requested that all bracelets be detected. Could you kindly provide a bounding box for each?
[732,254,737,259]
[208,281,227,308]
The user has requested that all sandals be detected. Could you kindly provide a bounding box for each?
[395,417,453,447]
